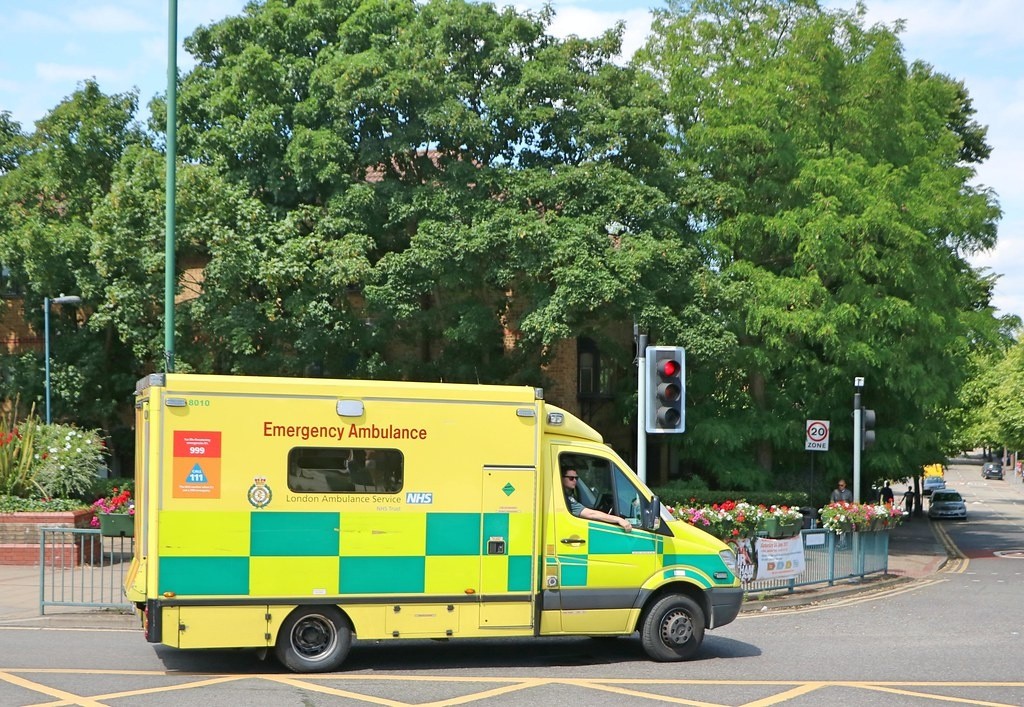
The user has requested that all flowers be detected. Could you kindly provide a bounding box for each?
[817,497,903,534]
[90,488,135,528]
[666,497,803,537]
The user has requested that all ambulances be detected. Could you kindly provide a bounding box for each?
[133,372,745,676]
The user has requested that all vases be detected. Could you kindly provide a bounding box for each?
[885,521,897,530]
[763,518,802,539]
[858,519,873,532]
[99,513,134,537]
[821,516,856,532]
[732,521,756,539]
[695,520,731,540]
[872,520,885,532]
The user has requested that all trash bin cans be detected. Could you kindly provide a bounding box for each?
[796,506,818,529]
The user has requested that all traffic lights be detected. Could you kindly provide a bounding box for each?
[860,406,877,453]
[646,345,686,434]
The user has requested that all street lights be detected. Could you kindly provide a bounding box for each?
[45,293,81,425]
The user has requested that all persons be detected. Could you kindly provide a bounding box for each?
[830,479,852,503]
[561,466,632,532]
[879,481,894,505]
[900,485,915,514]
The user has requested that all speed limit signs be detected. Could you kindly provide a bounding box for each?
[808,422,827,441]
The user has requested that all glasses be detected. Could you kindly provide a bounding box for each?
[563,475,578,481]
[837,484,845,486]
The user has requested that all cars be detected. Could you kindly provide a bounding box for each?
[924,476,947,495]
[927,489,967,520]
[980,461,1003,480]
